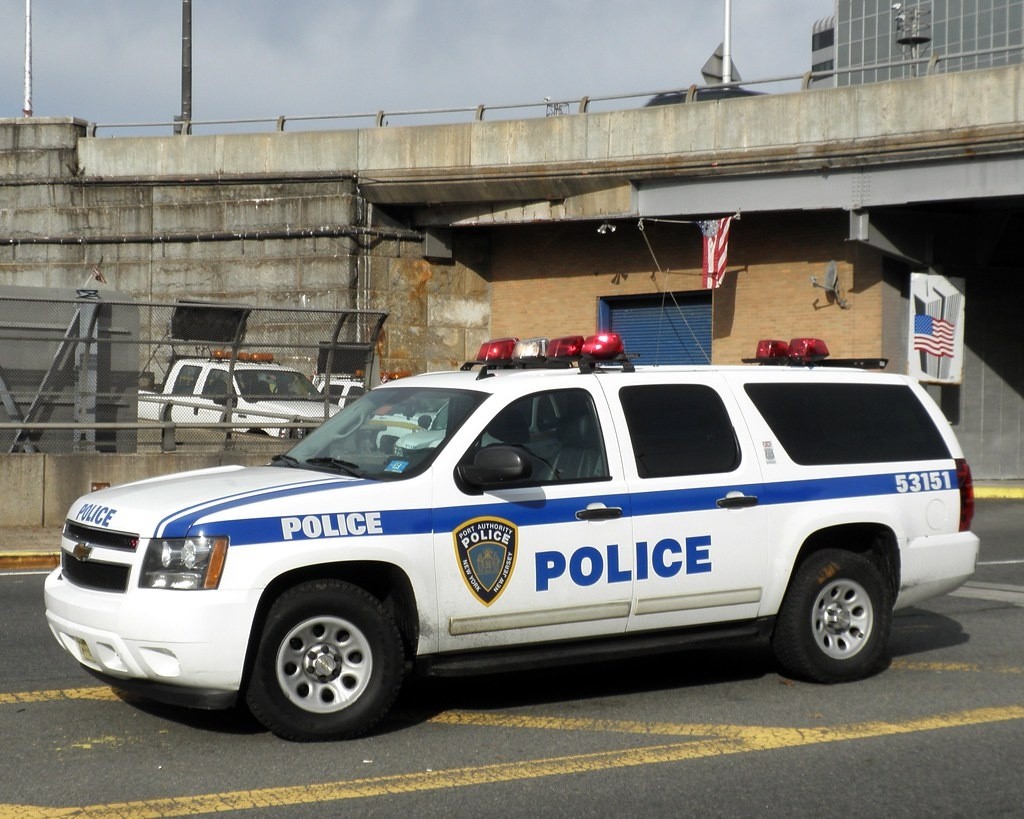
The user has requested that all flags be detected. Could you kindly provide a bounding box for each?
[697,214,732,289]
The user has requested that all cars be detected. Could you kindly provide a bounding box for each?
[311,371,438,456]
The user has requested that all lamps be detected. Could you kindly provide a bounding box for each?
[596,219,617,235]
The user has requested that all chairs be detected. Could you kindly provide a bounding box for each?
[535,410,605,480]
[487,409,531,449]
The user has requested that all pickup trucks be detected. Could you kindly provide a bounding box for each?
[135,349,346,442]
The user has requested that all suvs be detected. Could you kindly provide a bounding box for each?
[42,327,982,748]
